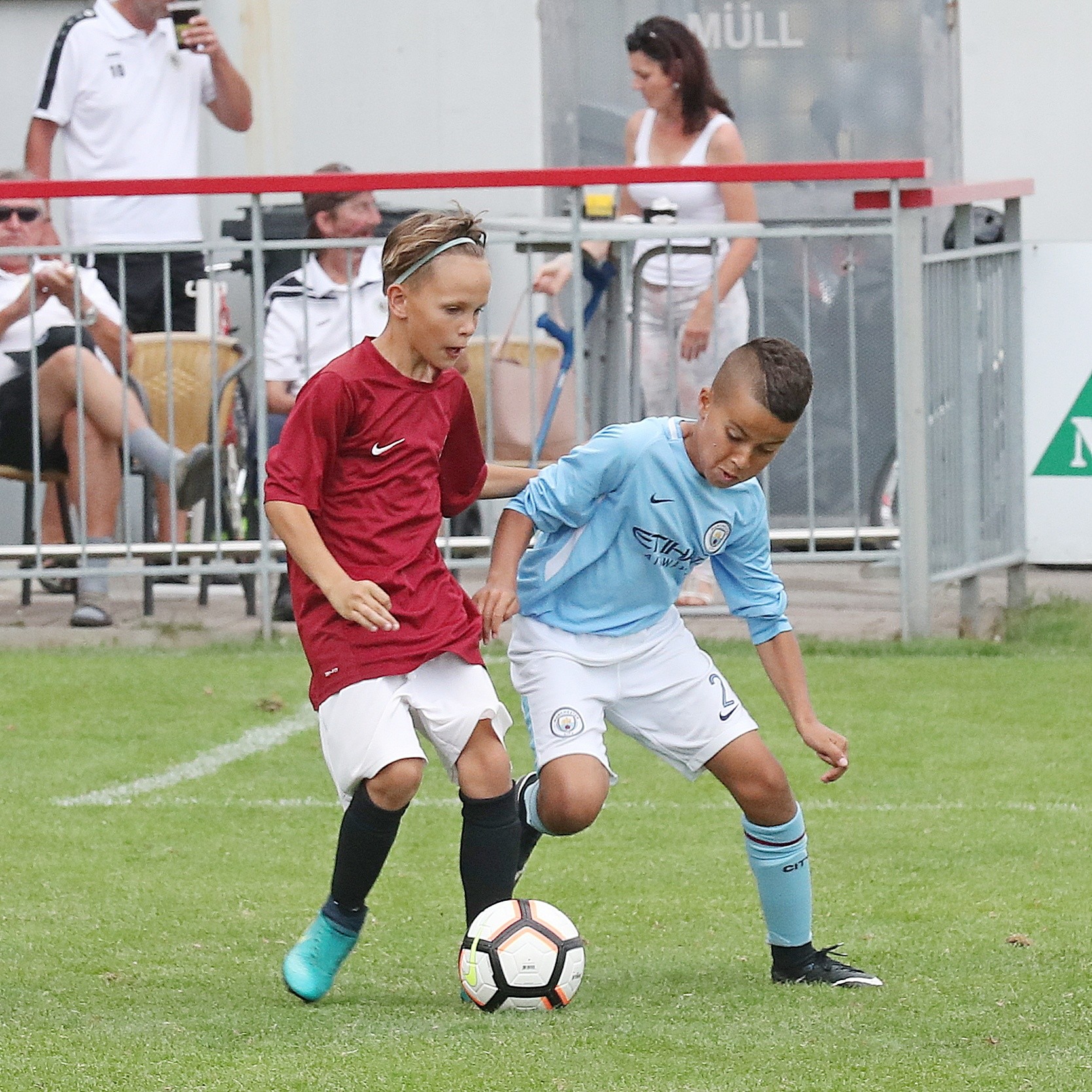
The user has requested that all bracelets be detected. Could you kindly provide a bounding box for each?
[81,305,97,329]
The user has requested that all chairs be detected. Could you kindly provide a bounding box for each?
[0,328,580,625]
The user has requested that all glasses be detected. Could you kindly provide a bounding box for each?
[636,28,677,62]
[0,206,41,222]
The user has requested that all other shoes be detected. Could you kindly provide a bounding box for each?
[70,590,112,626]
[177,442,227,510]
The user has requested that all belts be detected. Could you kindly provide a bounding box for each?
[643,282,677,293]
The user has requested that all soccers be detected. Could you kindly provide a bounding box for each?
[456,898,588,1013]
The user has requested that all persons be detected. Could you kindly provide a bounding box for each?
[23,0,251,589]
[264,198,543,1003]
[0,170,211,628]
[263,163,388,624]
[470,335,883,990]
[533,14,760,607]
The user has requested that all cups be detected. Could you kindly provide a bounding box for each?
[186,278,227,335]
[582,183,617,218]
[168,1,201,49]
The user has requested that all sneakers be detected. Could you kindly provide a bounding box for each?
[514,772,539,886]
[771,942,883,987]
[460,989,472,1002]
[283,911,359,1000]
